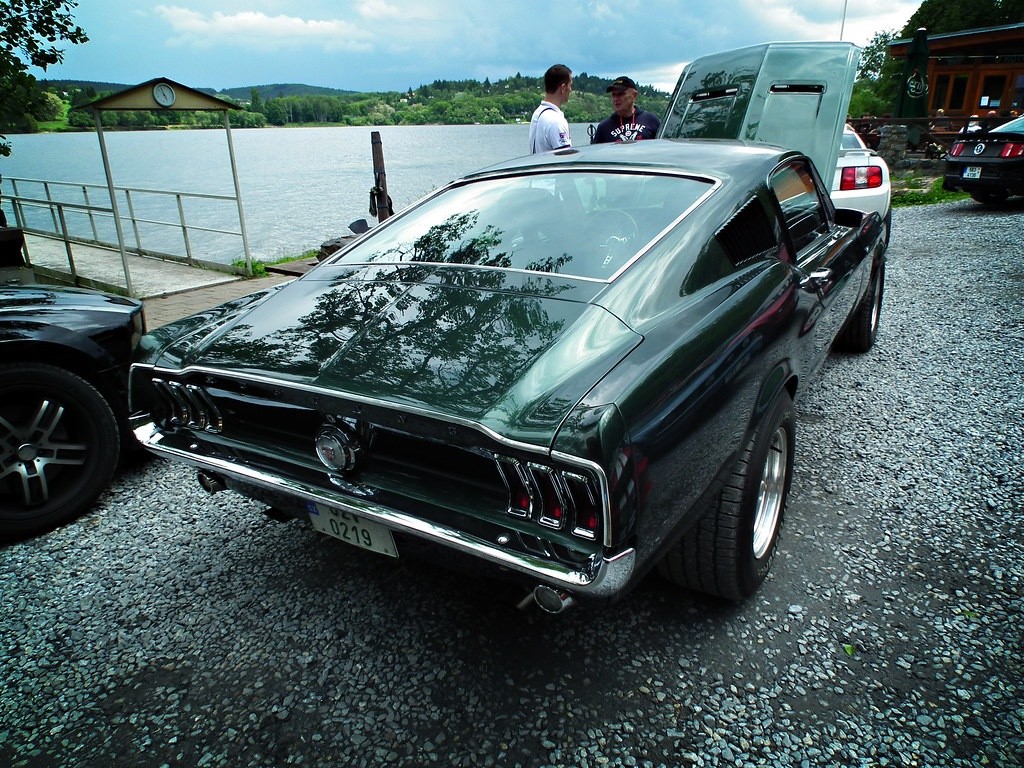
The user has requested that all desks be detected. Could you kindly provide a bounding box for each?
[920,132,960,160]
[857,133,880,148]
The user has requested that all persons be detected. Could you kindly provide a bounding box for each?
[953,110,1018,141]
[543,148,579,156]
[589,76,661,144]
[930,109,952,132]
[529,64,573,154]
[847,113,890,146]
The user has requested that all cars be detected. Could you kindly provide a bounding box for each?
[833,123,892,247]
[123,36,888,617]
[1,284,147,541]
[940,111,1024,204]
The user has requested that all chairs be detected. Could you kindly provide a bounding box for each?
[501,187,565,246]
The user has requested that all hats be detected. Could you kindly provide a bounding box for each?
[969,114,979,126]
[606,76,635,92]
[988,111,996,116]
